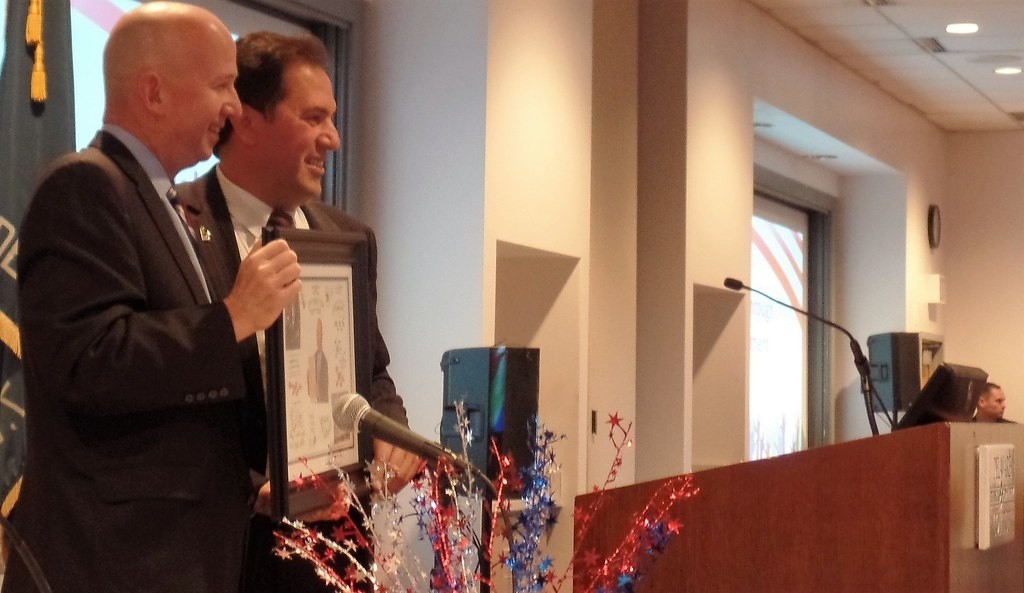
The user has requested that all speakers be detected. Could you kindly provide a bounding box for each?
[440,346,541,500]
[867,332,921,412]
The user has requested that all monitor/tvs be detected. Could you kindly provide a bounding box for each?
[896,363,988,430]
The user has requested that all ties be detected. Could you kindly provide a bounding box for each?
[166,181,218,307]
[267,208,296,228]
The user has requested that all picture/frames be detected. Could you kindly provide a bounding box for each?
[261,227,376,516]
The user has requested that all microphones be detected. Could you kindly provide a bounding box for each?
[724,277,872,388]
[332,393,480,476]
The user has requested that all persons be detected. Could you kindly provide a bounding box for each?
[174,30,422,593]
[971,383,1017,424]
[15,0,306,593]
[316,318,327,401]
